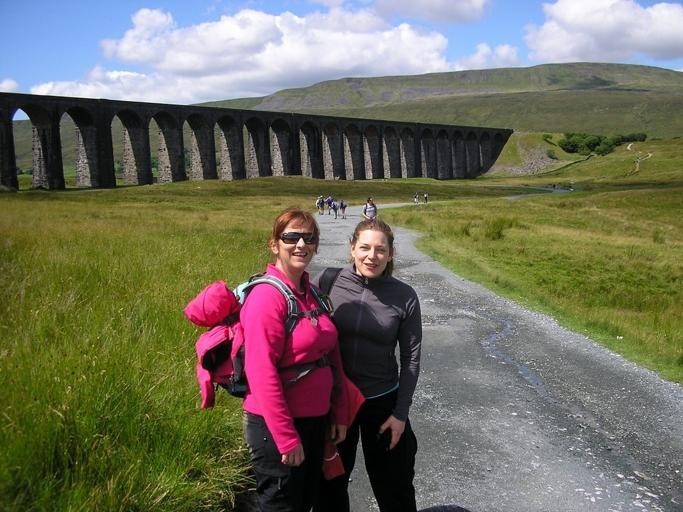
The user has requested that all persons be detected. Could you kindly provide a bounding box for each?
[360,196,377,220]
[324,195,334,215]
[423,191,428,204]
[317,195,324,215]
[315,196,320,214]
[413,191,418,205]
[331,198,339,219]
[339,199,346,219]
[236,206,351,511]
[310,217,424,512]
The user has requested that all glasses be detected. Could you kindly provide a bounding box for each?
[278,232,315,245]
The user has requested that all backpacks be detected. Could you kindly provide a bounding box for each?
[208,271,332,398]
